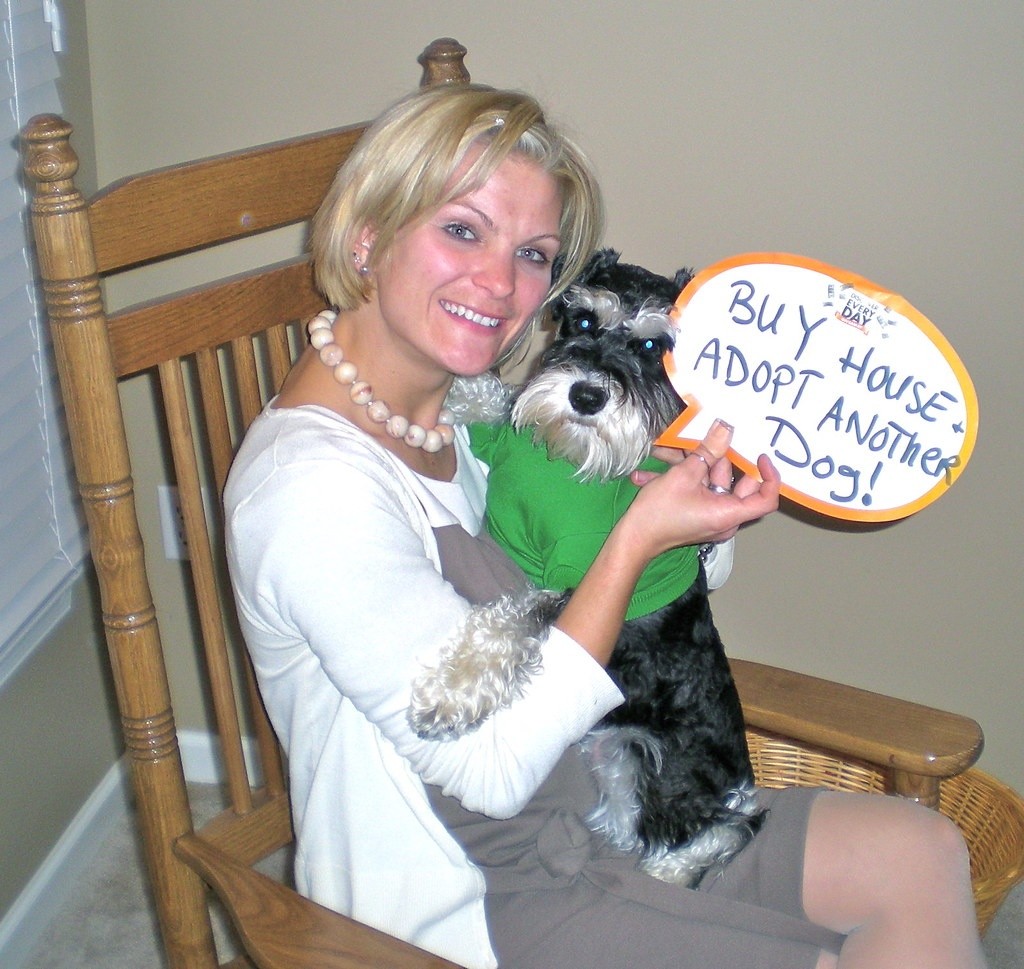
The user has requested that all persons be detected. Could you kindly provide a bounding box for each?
[223,82,991,969]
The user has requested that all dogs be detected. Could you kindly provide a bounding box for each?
[405,244,769,891]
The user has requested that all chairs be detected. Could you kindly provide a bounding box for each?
[18,37,984,969]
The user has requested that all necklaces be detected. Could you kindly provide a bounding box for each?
[307,307,456,451]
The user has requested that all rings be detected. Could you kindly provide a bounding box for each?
[707,481,733,494]
[690,451,712,479]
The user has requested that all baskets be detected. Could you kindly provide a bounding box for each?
[744,725,1024,938]
